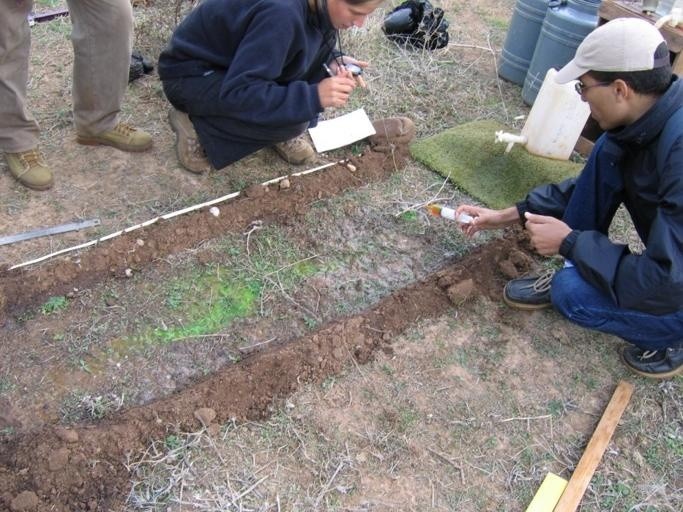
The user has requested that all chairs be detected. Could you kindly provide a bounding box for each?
[167,107,212,174]
[273,137,316,165]
[75,121,153,152]
[3,146,54,190]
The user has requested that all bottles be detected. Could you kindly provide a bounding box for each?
[553,16,671,87]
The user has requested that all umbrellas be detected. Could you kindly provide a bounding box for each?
[503,269,556,311]
[619,343,683,379]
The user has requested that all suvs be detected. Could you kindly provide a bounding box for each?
[521,0,602,108]
[498,0,550,86]
[523,68,592,162]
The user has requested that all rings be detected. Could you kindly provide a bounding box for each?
[323,63,352,102]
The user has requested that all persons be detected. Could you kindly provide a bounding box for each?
[157,0,382,174]
[0,0,154,192]
[151,0,153,0]
[452,16,682,380]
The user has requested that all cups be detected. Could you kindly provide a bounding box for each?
[575,79,611,97]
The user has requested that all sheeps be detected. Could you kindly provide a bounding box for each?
[494,128,525,156]
